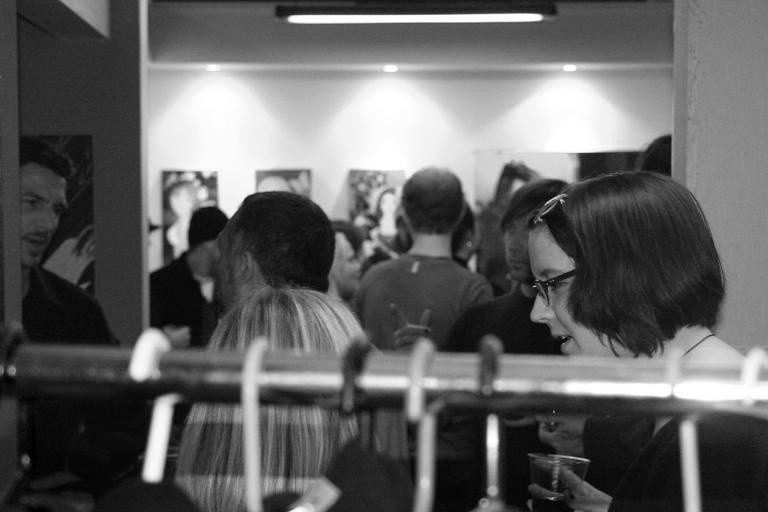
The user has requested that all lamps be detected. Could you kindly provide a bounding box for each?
[274,1,557,25]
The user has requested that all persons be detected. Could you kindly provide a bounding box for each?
[452,199,482,270]
[217,190,334,320]
[164,173,209,260]
[151,203,228,345]
[351,169,388,227]
[348,167,495,351]
[364,188,401,261]
[387,178,570,510]
[526,170,766,512]
[18,139,149,509]
[173,288,411,512]
[639,132,671,181]
[360,195,413,273]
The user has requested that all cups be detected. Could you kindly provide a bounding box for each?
[526,451,591,512]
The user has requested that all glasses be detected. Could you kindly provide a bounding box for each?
[534,269,575,306]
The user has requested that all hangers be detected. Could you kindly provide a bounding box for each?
[404,336,442,510]
[479,334,506,512]
[664,351,701,512]
[2,321,34,475]
[336,339,373,454]
[129,327,179,483]
[738,347,767,409]
[240,334,273,512]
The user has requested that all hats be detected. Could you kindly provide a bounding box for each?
[189,205,228,247]
